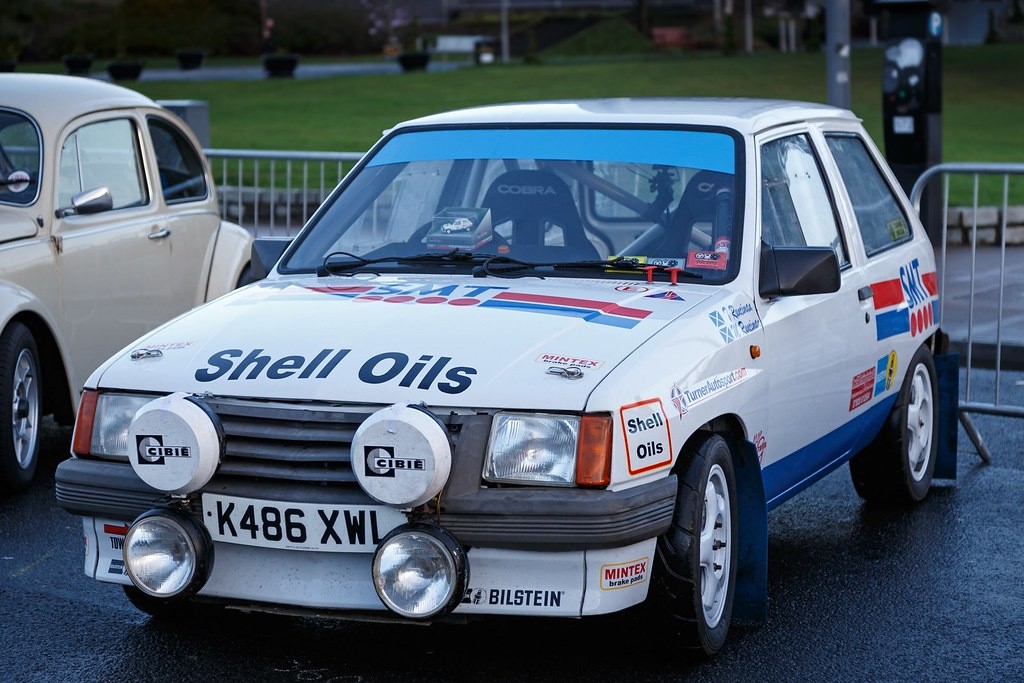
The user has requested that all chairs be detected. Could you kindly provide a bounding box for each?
[479,169,602,264]
[642,170,785,261]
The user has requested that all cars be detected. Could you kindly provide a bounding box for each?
[0,72,254,491]
[55,99,951,661]
